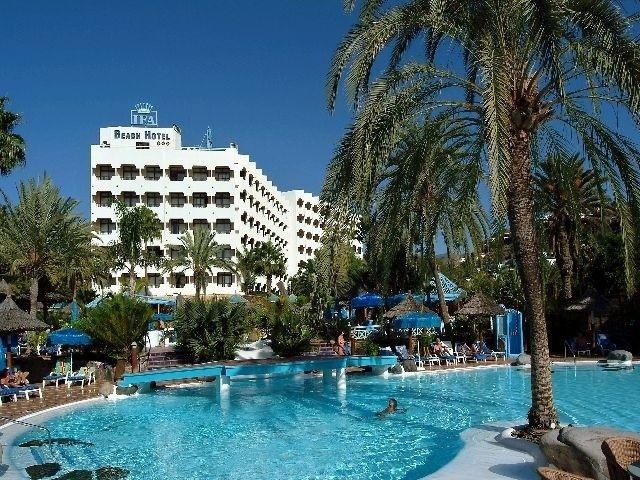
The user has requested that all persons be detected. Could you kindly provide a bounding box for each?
[468,343,478,355]
[379,399,398,417]
[1,368,29,388]
[459,342,470,351]
[576,332,588,348]
[338,331,345,356]
[433,336,448,356]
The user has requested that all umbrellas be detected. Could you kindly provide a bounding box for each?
[394,312,440,367]
[564,296,619,348]
[0,295,50,367]
[350,292,383,320]
[386,290,436,354]
[48,328,91,376]
[453,286,507,352]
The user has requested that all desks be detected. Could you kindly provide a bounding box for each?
[627,461,640,480]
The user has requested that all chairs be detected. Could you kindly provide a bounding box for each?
[602,435,640,480]
[535,466,595,480]
[564,331,616,359]
[1,366,98,405]
[382,344,507,371]
